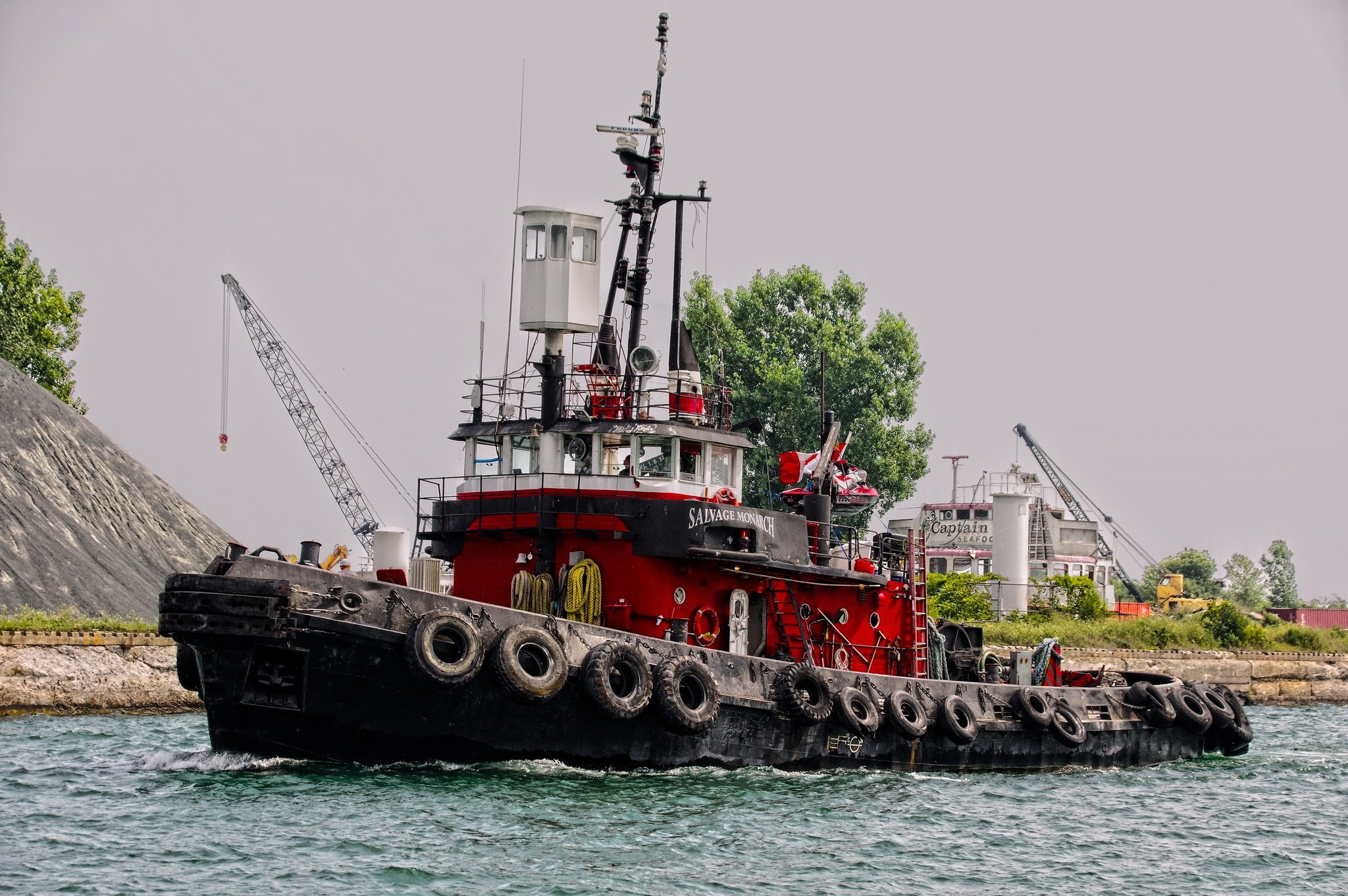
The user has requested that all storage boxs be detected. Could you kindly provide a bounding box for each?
[1267,608,1348,630]
[1115,602,1150,619]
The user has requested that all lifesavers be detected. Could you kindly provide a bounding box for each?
[1182,679,1210,688]
[580,641,653,720]
[1009,688,1051,729]
[1212,685,1253,751]
[693,605,721,646]
[937,695,978,746]
[884,690,927,740]
[713,488,739,506]
[1218,744,1249,757]
[1127,681,1179,729]
[1167,689,1213,734]
[773,662,834,727]
[653,656,721,736]
[1048,700,1086,749]
[835,687,878,742]
[1190,685,1235,729]
[490,623,569,704]
[404,610,486,690]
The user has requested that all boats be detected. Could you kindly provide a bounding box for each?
[155,12,1261,770]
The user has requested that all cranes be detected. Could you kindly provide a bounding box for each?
[1011,423,1228,624]
[218,271,433,564]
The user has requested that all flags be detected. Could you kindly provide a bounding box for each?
[780,443,847,484]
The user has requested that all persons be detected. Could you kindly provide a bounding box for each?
[618,455,630,476]
[339,559,357,577]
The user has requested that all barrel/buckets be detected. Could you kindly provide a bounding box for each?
[891,571,907,583]
